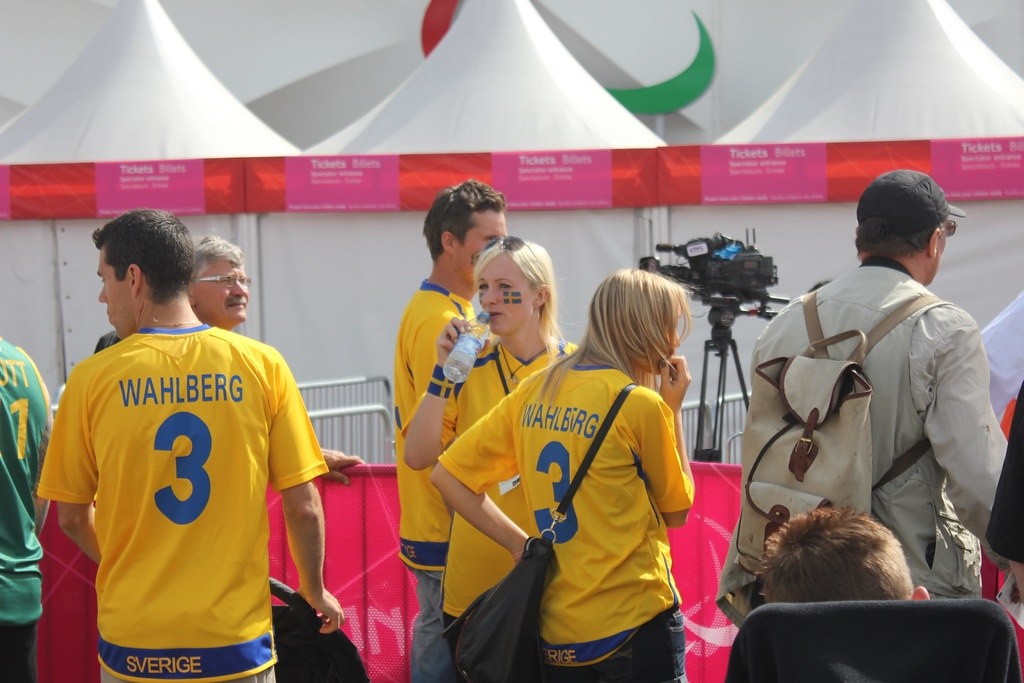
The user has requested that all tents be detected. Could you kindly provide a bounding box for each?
[1,0,1024,465]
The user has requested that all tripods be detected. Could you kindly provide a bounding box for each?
[694,296,750,464]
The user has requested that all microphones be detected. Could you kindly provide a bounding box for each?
[656,243,681,254]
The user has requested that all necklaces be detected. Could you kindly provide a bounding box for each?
[500,342,549,384]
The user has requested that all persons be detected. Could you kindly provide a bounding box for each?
[985,380,1024,604]
[394,177,507,683]
[715,169,1008,630]
[36,210,345,683]
[0,336,54,683]
[761,503,931,602]
[400,235,581,683]
[432,268,695,683]
[190,235,365,486]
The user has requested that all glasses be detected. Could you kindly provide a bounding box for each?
[933,220,958,237]
[484,235,537,262]
[190,275,251,289]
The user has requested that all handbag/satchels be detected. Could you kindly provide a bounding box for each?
[442,536,561,682]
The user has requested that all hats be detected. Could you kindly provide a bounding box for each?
[856,169,967,234]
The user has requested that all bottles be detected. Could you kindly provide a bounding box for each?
[443,311,490,383]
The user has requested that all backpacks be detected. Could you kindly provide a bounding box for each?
[735,290,944,580]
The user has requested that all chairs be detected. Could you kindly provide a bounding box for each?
[269,576,370,683]
[723,599,1022,683]
